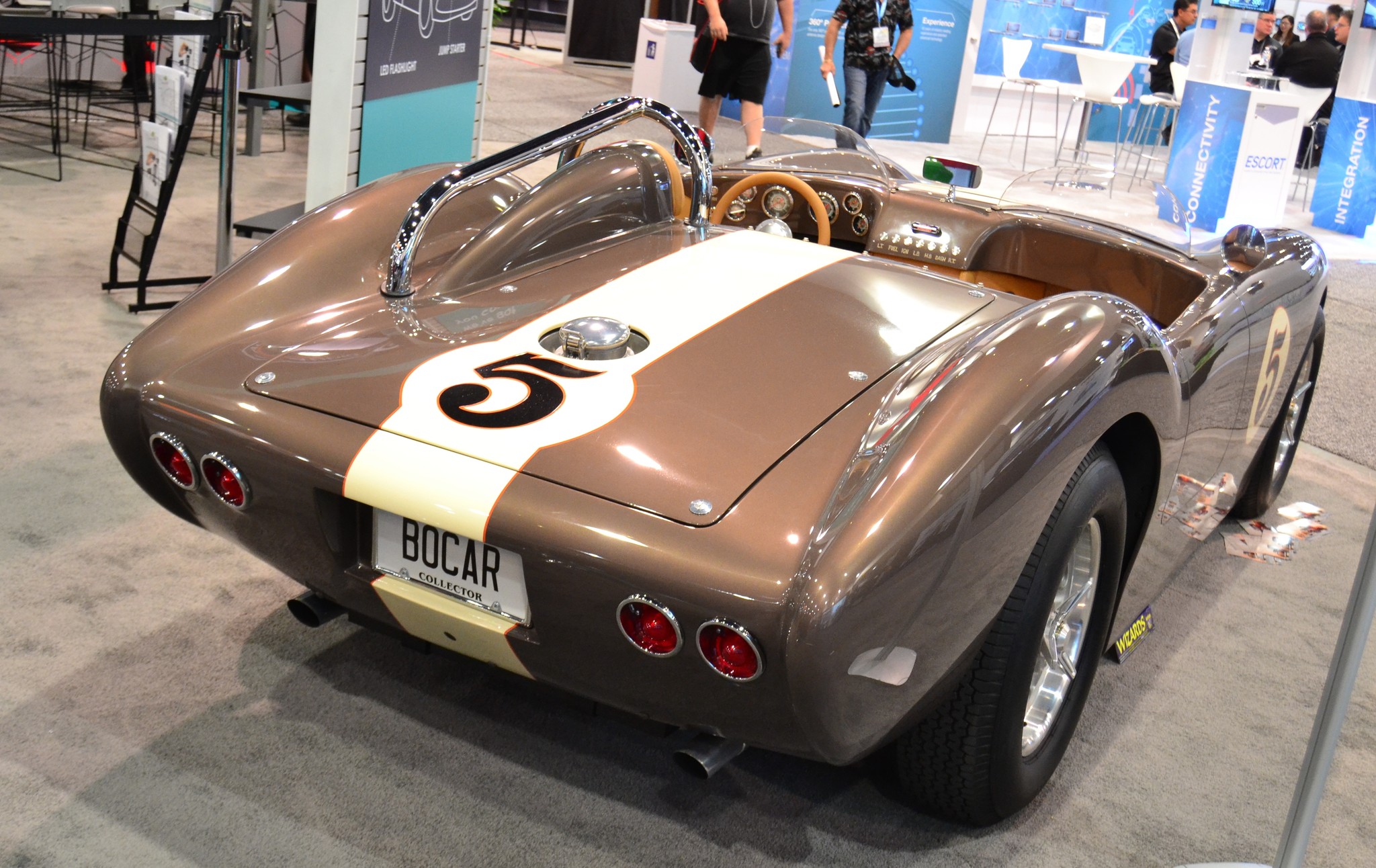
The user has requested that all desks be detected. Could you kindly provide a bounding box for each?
[1042,42,1159,199]
[1244,67,1291,92]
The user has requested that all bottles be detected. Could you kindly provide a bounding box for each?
[1258,47,1270,66]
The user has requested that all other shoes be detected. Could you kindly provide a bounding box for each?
[744,145,764,160]
[1296,146,1323,170]
[1161,126,1171,148]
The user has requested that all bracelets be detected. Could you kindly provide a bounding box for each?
[823,59,832,63]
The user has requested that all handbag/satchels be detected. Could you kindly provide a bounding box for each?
[690,0,720,74]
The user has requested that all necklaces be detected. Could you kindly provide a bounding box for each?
[750,1,768,27]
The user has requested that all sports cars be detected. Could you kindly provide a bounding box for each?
[98,93,1337,831]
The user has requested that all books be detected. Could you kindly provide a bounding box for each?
[139,8,207,210]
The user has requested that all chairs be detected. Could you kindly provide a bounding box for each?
[1114,60,1189,195]
[1,0,287,182]
[1051,51,1136,199]
[977,37,1061,173]
[1278,77,1333,211]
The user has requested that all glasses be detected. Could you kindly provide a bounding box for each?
[1259,18,1277,25]
[1281,21,1290,26]
[1334,23,1344,28]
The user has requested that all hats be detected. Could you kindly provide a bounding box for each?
[888,54,916,92]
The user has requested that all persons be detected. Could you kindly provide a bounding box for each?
[1149,0,1200,147]
[1273,11,1341,168]
[690,0,795,167]
[1323,5,1344,49]
[1271,14,1302,49]
[819,0,914,150]
[1245,10,1283,91]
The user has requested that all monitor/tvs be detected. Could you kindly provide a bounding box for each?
[1210,0,1276,15]
[1360,0,1376,30]
